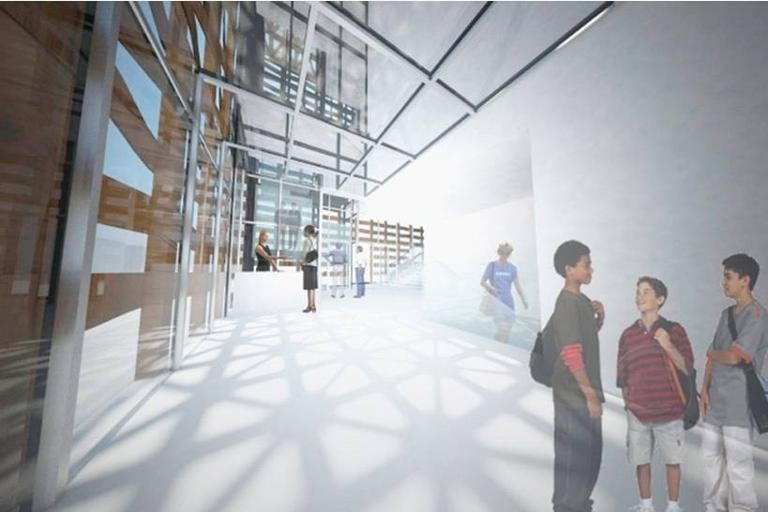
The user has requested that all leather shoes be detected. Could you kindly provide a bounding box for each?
[302,305,317,314]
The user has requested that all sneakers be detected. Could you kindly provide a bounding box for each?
[330,293,365,299]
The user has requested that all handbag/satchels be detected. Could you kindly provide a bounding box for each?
[306,248,319,263]
[529,326,556,385]
[675,366,701,432]
[745,373,767,435]
